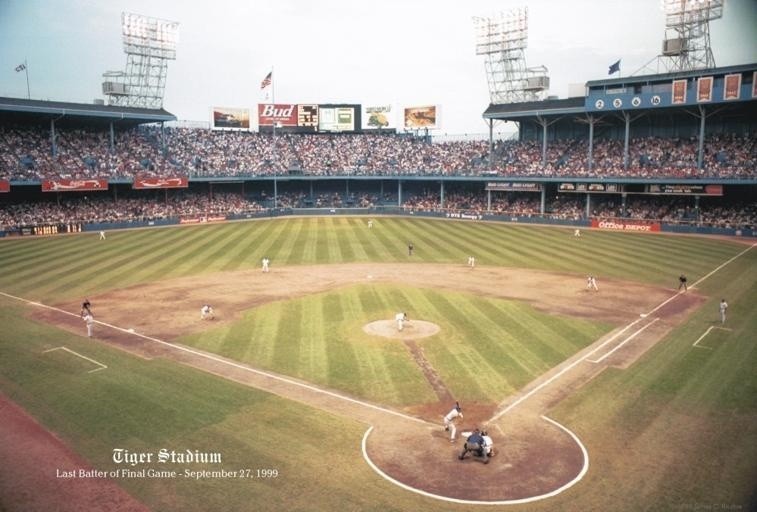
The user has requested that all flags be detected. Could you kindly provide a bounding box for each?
[608,59,620,75]
[14,63,25,73]
[259,72,271,91]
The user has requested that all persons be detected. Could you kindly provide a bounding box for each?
[467,255,474,267]
[261,255,268,273]
[80,312,94,338]
[395,311,407,331]
[677,273,687,292]
[479,429,494,457]
[78,298,92,319]
[199,304,215,320]
[443,402,463,443]
[408,242,412,256]
[458,427,489,464]
[1,111,756,239]
[584,275,598,291]
[717,297,727,325]
